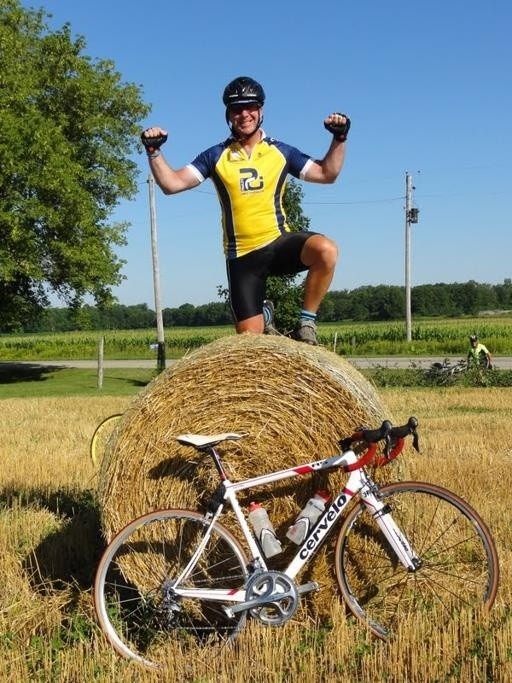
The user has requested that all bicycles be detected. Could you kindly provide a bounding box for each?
[95,416,499,679]
[90,412,123,468]
[429,357,467,386]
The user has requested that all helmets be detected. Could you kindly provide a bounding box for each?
[223,76,265,108]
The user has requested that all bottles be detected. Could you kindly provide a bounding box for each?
[249,488,332,560]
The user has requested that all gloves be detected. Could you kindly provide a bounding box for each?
[142,127,168,152]
[322,112,351,140]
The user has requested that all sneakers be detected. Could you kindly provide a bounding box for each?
[262,299,278,334]
[291,318,320,345]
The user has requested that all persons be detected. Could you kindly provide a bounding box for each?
[464,333,494,369]
[142,75,353,348]
